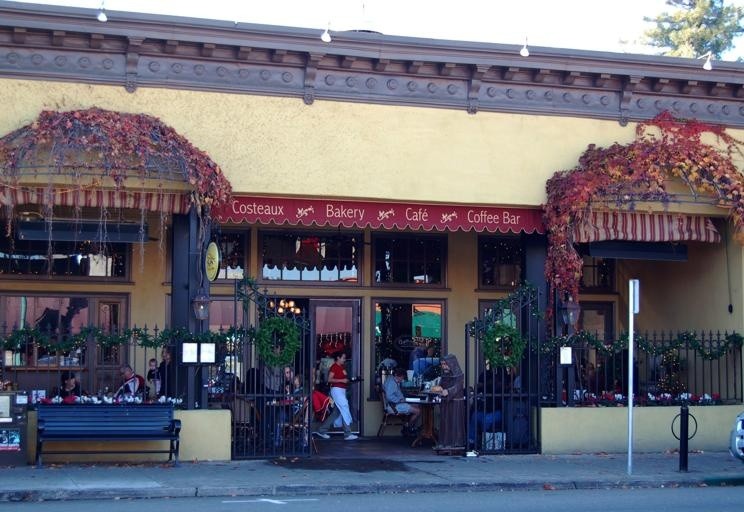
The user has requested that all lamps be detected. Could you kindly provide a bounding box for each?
[520,35,532,56]
[699,51,714,70]
[321,23,333,41]
[191,285,212,321]
[96,2,109,21]
[561,289,581,327]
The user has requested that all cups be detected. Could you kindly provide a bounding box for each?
[405,369,414,382]
[4,350,12,366]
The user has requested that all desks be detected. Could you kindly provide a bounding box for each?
[406,396,444,446]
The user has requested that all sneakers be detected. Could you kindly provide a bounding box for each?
[401,425,417,438]
[344,435,359,440]
[317,433,330,438]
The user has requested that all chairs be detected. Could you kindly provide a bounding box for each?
[216,388,323,452]
[378,391,412,441]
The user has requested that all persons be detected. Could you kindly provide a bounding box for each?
[466,366,510,450]
[430,354,466,447]
[269,351,358,451]
[383,367,422,435]
[108,345,172,399]
[50,372,90,399]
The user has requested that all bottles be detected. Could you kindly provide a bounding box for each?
[12,341,21,367]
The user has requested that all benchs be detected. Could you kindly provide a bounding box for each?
[36,402,181,468]
